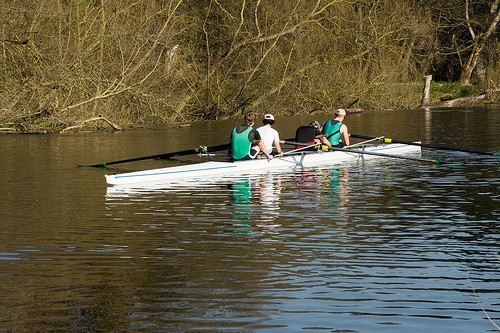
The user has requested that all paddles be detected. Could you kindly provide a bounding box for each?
[77,144,228,166]
[154,144,230,159]
[348,133,500,157]
[279,140,463,165]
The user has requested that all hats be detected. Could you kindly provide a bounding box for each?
[262,113,275,120]
[336,108,347,116]
[309,120,321,128]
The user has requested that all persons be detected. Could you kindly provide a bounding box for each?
[297,168,321,224]
[322,162,350,227]
[255,174,282,241]
[226,179,253,242]
[320,108,349,151]
[228,111,273,163]
[295,121,331,155]
[256,114,282,158]
